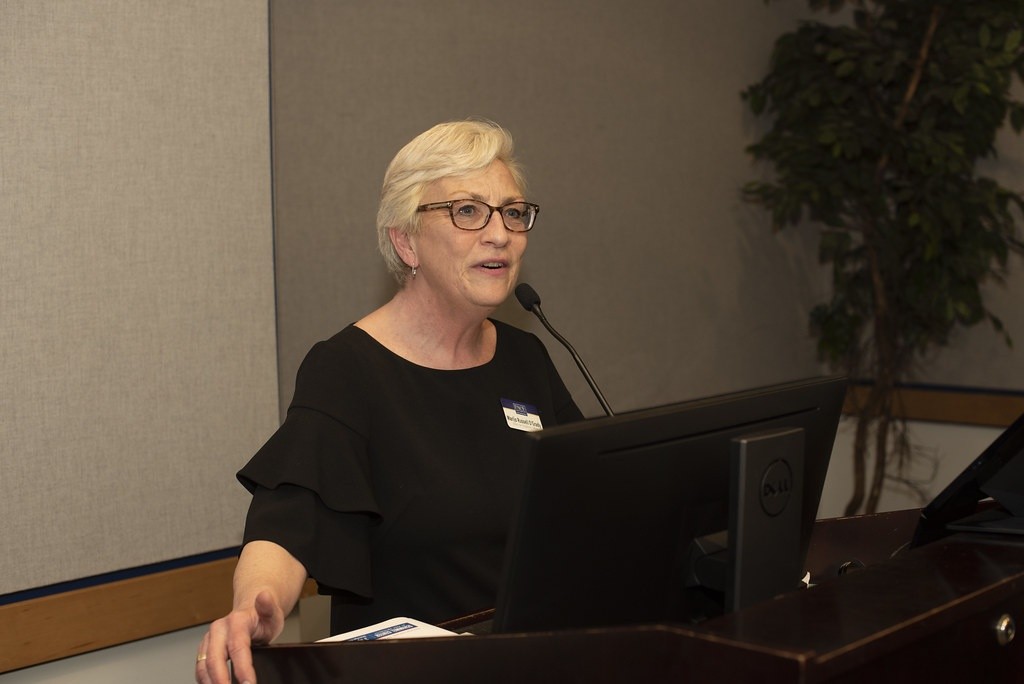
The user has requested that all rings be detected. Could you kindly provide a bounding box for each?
[196,654,206,664]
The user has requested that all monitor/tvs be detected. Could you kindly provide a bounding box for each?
[497,375,853,634]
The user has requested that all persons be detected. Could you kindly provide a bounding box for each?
[195,122,585,684]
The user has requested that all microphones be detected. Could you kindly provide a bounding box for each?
[515,282,612,417]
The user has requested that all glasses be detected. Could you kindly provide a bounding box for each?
[416,198,540,233]
